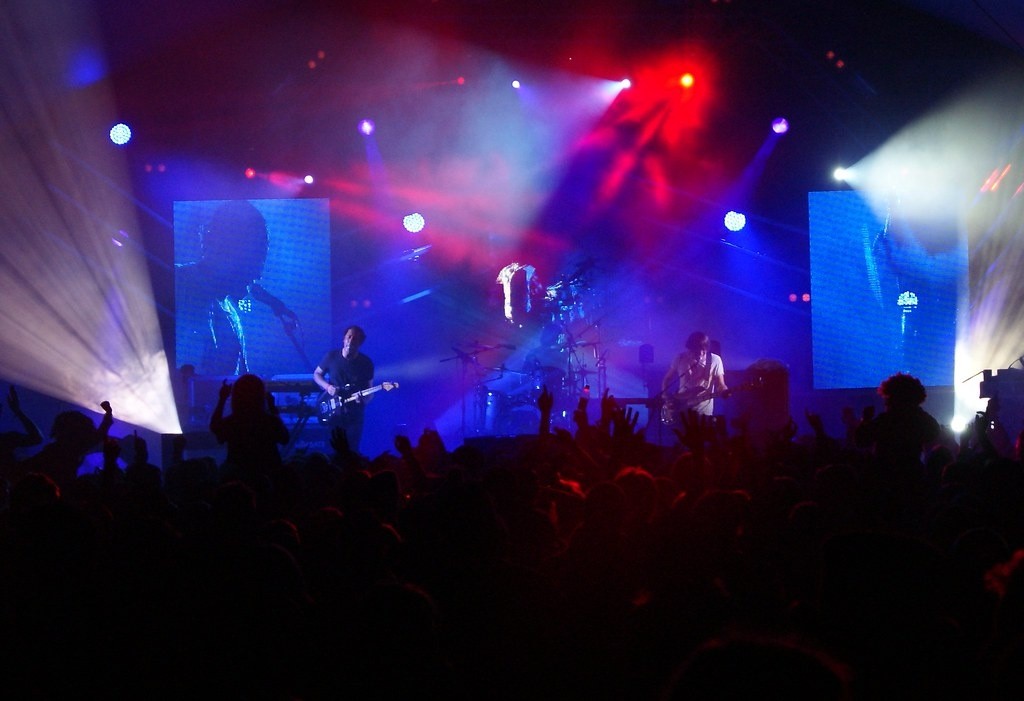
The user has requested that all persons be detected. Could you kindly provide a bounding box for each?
[519,322,578,385]
[208,373,286,491]
[856,371,937,464]
[3,393,1020,699]
[173,201,267,373]
[314,324,374,475]
[661,330,729,428]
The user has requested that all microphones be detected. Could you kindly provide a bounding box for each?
[498,344,516,349]
[696,357,702,365]
[248,282,296,322]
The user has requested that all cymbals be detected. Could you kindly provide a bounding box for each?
[568,369,597,375]
[552,340,587,350]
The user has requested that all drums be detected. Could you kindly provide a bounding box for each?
[495,403,543,434]
[532,366,566,397]
[478,390,504,433]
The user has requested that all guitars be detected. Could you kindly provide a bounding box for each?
[316,381,400,427]
[661,376,764,424]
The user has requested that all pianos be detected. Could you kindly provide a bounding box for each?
[266,375,331,457]
